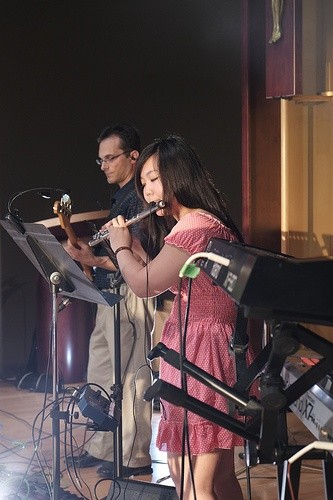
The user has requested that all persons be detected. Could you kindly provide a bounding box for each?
[63,126,177,477]
[99,136,261,500]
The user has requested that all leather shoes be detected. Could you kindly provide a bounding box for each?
[63,451,105,468]
[96,461,154,478]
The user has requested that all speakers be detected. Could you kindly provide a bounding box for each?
[107,477,179,500]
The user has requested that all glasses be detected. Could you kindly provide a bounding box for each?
[95,149,133,166]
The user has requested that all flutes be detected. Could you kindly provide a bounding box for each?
[87,199,167,248]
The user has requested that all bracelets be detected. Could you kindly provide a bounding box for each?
[114,246,133,258]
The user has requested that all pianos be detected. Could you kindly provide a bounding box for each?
[195,236,333,327]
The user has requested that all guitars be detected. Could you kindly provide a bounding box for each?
[52,194,95,286]
